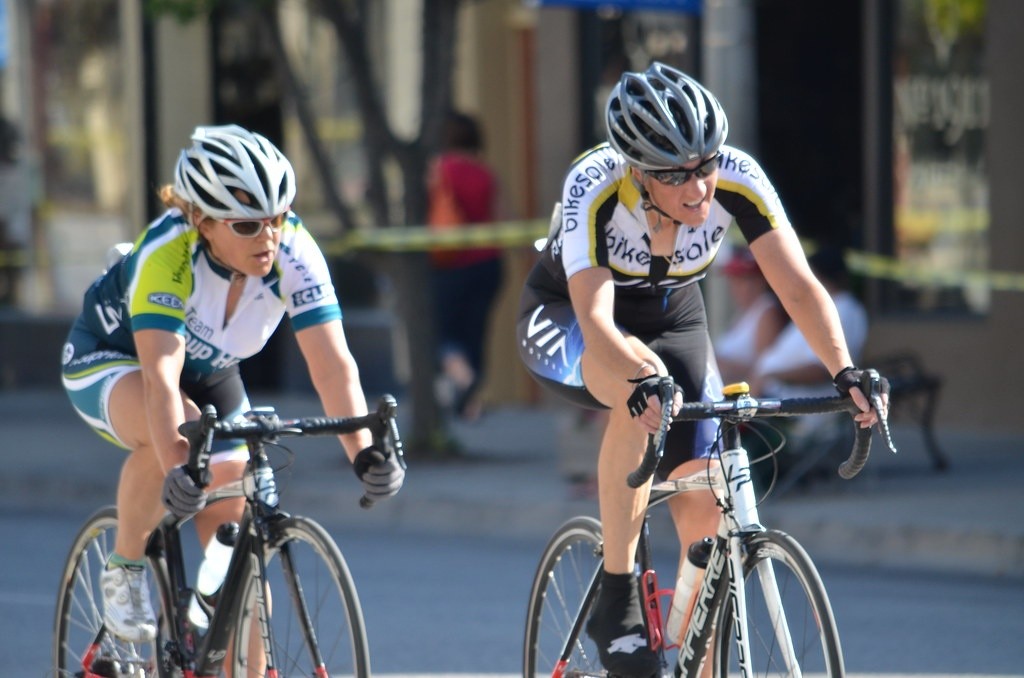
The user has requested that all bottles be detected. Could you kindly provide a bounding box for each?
[186,521,240,630]
[667,537,715,646]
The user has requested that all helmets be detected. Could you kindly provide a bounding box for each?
[604,63,728,171]
[172,126,297,221]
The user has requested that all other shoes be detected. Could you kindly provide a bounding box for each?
[585,591,659,678]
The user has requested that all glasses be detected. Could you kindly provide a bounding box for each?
[214,208,290,239]
[646,150,724,186]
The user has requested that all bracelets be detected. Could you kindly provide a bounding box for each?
[630,364,650,388]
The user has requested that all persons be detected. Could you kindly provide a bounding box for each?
[708,236,870,493]
[58,122,405,678]
[510,57,892,677]
[419,105,504,460]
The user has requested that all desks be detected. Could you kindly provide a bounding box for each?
[347,220,552,464]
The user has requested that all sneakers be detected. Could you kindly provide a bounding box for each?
[100,554,159,642]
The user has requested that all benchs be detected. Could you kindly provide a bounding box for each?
[733,350,946,492]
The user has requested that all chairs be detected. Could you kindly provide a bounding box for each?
[0,199,61,314]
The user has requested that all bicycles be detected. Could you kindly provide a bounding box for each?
[522,366,899,678]
[51,394,407,678]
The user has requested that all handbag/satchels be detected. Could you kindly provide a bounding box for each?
[425,156,463,265]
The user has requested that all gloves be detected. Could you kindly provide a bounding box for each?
[355,444,406,502]
[160,464,207,518]
